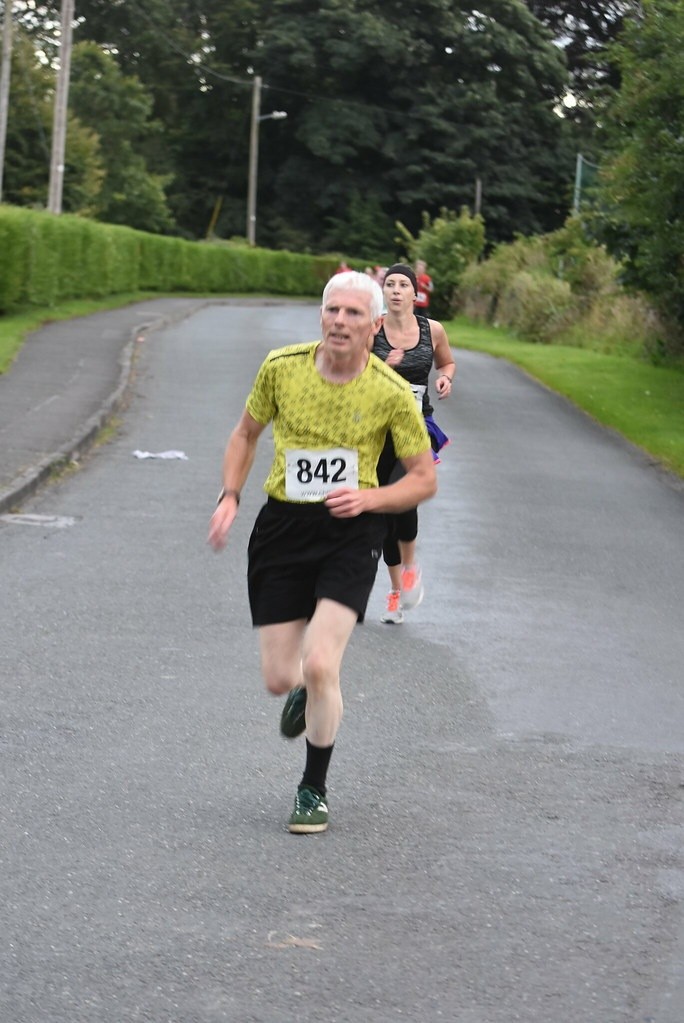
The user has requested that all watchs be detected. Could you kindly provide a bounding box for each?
[214,486,241,507]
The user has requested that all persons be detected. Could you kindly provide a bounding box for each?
[206,270,439,833]
[365,262,456,624]
[335,257,433,319]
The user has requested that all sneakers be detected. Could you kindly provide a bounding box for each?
[382,589,403,624]
[279,685,307,738]
[290,783,330,834]
[399,562,424,610]
